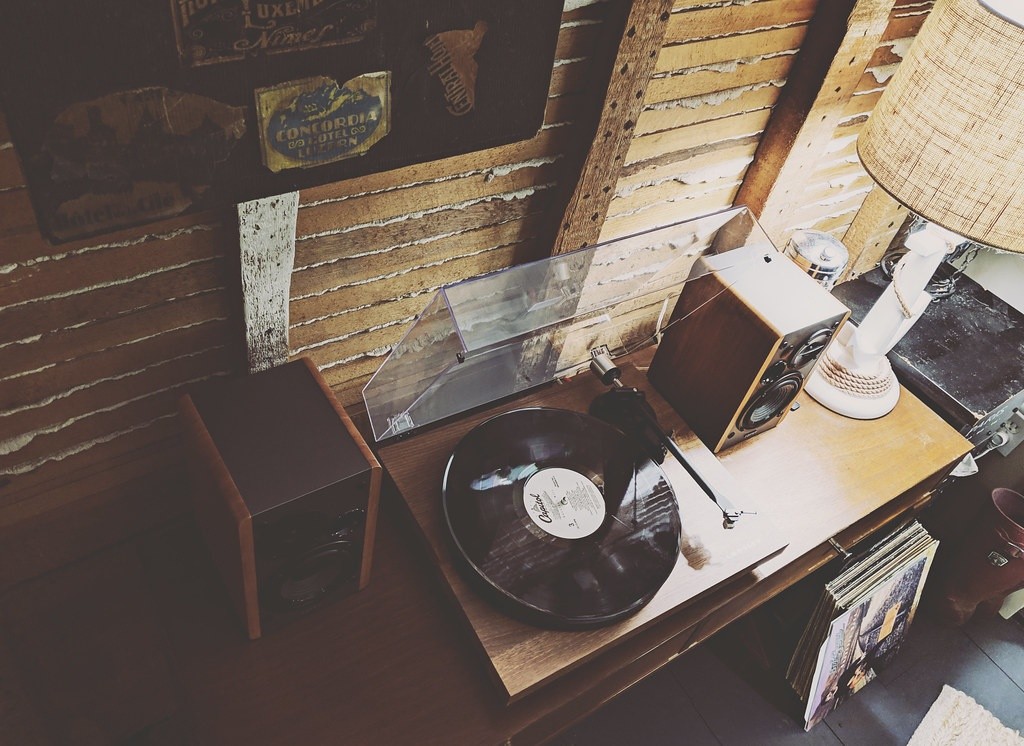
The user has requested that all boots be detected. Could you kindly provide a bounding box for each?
[920,488,1024,625]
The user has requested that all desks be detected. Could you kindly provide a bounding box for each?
[0,343,976,746]
[829,257,1024,504]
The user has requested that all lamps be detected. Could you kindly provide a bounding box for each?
[804,0,1024,420]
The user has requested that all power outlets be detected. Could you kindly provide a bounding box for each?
[995,410,1024,457]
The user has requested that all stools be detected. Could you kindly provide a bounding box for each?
[176,356,383,640]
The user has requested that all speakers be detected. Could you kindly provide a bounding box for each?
[646,241,850,454]
